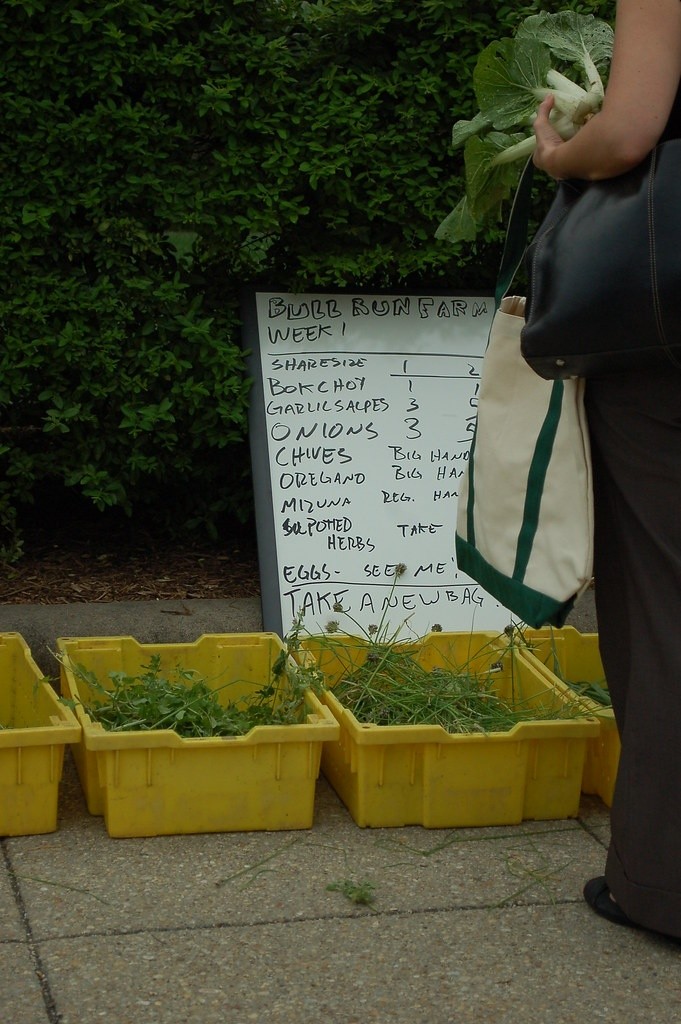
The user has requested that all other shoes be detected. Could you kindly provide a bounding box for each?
[584,876,681,948]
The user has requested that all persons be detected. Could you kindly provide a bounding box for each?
[530,1,681,955]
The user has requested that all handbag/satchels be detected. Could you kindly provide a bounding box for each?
[453,162,594,628]
[521,137,681,381]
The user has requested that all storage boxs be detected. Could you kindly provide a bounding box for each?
[0,628,85,838]
[513,620,635,808]
[53,629,344,840]
[285,628,607,833]
